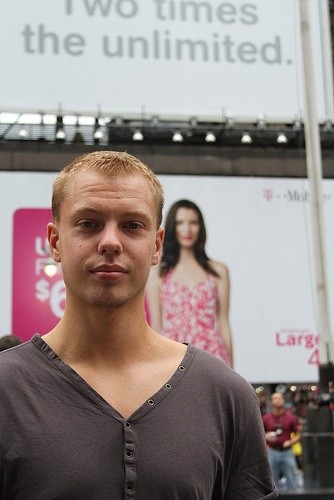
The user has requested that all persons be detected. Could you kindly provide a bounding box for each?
[255,384,333,486]
[145,199,232,368]
[262,391,303,492]
[0,150,279,500]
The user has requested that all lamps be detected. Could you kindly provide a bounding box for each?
[17,128,289,145]
